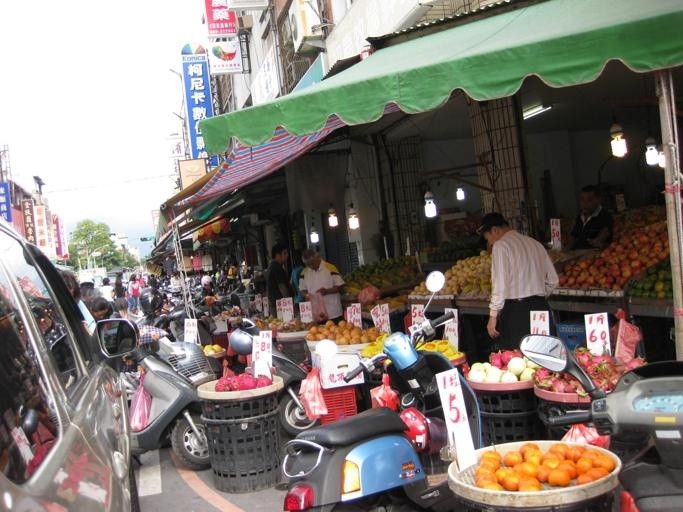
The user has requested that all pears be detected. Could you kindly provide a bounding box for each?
[410,250,492,297]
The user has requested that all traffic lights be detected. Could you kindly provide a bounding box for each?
[140,236,152,243]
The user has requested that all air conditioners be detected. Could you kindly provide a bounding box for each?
[285,0,325,55]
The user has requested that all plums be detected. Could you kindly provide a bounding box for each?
[477,443,616,492]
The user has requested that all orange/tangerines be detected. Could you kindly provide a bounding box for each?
[628,257,673,299]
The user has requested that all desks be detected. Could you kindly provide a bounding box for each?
[623,289,676,360]
[544,293,628,347]
[406,294,453,317]
[355,305,410,337]
[454,296,494,318]
[341,280,422,304]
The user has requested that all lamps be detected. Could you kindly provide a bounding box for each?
[455,180,465,201]
[327,180,338,227]
[347,168,359,230]
[608,116,628,160]
[521,101,553,122]
[644,133,661,166]
[423,185,437,219]
[309,215,319,244]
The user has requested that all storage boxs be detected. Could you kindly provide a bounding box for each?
[310,339,365,390]
[556,322,587,350]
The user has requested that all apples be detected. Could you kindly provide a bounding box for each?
[560,219,668,290]
[306,319,386,344]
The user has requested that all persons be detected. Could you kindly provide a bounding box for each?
[476,212,559,351]
[56,243,346,326]
[561,185,613,251]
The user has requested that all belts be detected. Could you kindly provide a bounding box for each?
[505,295,545,305]
